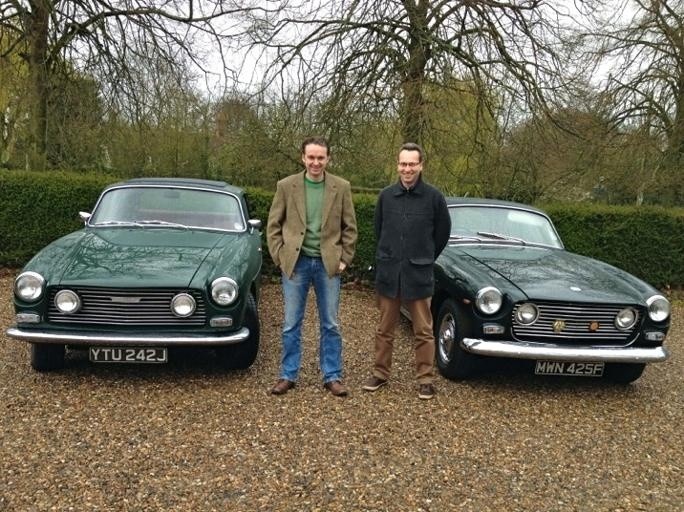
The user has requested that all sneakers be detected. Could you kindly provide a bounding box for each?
[418,384,433,400]
[362,378,387,391]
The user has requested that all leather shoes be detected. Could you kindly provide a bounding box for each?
[324,381,347,395]
[272,381,293,395]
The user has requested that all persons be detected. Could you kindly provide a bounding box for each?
[360,143,452,400]
[265,136,358,396]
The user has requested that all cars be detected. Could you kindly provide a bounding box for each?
[400,194,674,389]
[2,175,266,377]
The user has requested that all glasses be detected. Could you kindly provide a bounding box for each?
[398,162,420,167]
